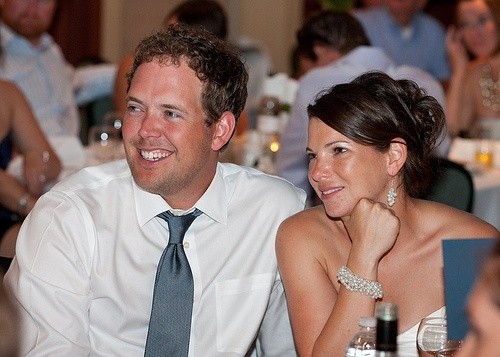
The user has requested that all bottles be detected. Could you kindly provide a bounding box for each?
[376,302,399,357]
[345,316,376,357]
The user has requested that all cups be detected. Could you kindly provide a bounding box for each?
[90,126,115,159]
[473,139,492,167]
[103,110,124,135]
[416,318,463,357]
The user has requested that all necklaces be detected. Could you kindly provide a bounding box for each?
[478,58,500,112]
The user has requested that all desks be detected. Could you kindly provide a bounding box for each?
[448,137,500,231]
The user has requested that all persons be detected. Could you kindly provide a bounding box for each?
[0,26,306,357]
[273,72,500,357]
[453,237,500,357]
[0,0,499,265]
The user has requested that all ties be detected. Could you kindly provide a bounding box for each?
[143,208,204,357]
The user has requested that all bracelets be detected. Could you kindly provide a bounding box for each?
[16,191,31,213]
[336,265,383,300]
